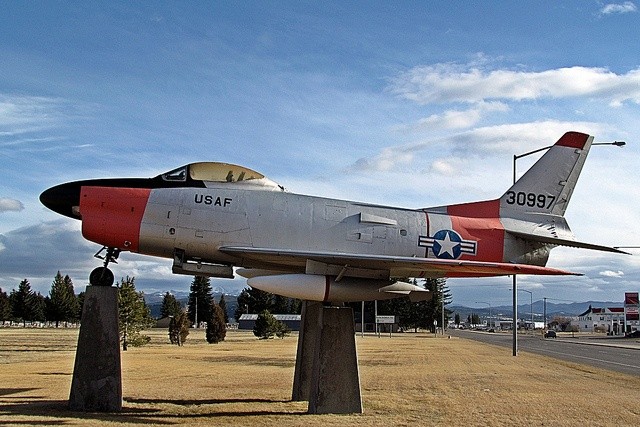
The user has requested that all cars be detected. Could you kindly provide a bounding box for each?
[543,330,556,337]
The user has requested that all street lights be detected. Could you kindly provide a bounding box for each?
[475,301,492,326]
[509,288,533,320]
[512,141,627,354]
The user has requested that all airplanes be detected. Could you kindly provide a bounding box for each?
[40,131,639,305]
